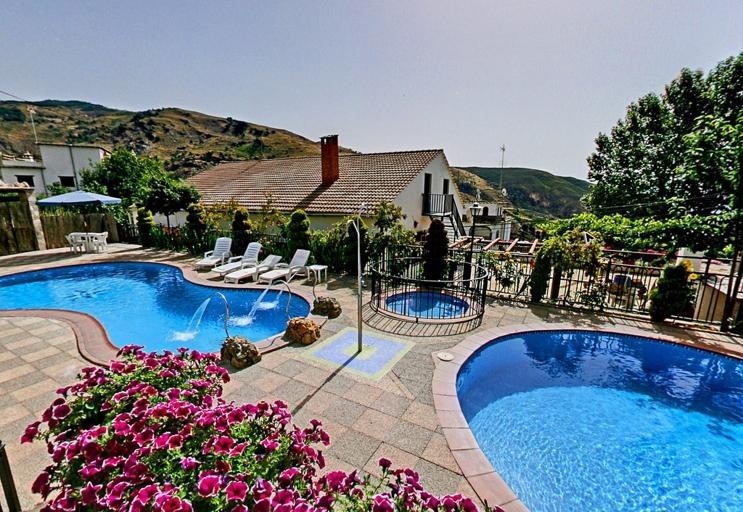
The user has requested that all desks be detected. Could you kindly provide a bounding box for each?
[307,265,328,283]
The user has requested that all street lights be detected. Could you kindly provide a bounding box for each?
[343,198,372,353]
[469,199,481,265]
[28,108,40,143]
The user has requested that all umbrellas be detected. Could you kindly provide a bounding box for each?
[36,190,122,232]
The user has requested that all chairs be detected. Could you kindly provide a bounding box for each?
[224,254,282,283]
[195,237,232,270]
[211,242,262,277]
[258,249,310,286]
[65,231,108,254]
